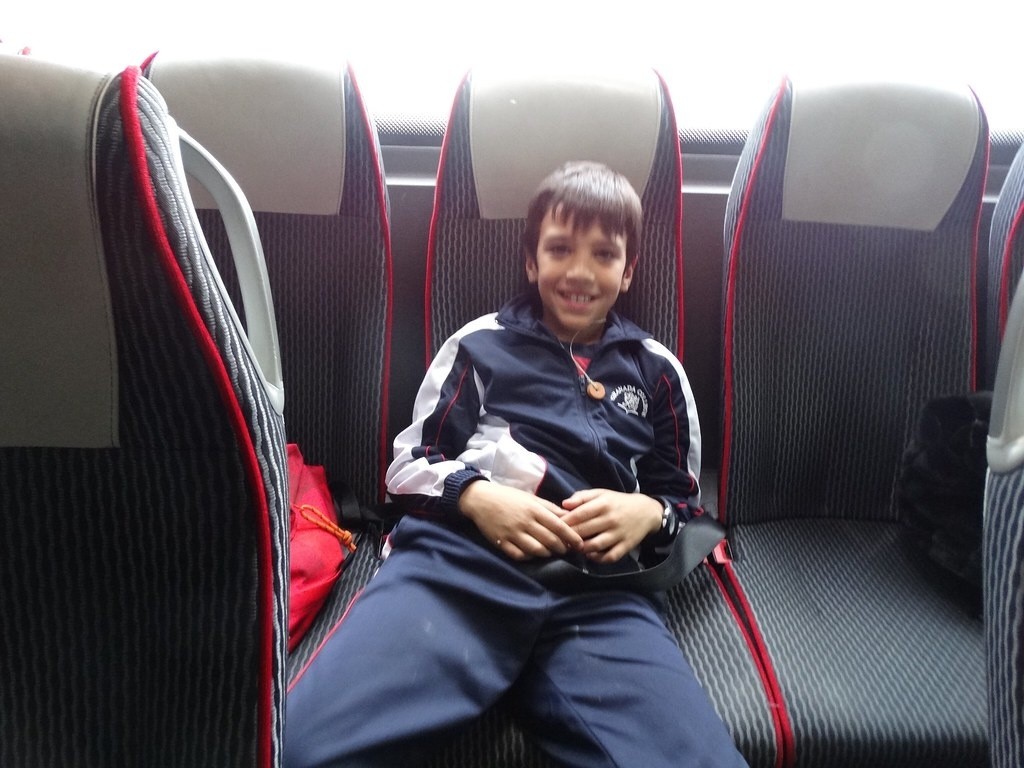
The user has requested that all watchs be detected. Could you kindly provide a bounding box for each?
[648,494,678,546]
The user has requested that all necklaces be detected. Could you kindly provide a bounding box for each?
[559,318,606,399]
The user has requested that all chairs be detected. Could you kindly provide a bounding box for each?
[0,44,1024,768]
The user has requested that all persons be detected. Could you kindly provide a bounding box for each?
[281,160,752,768]
